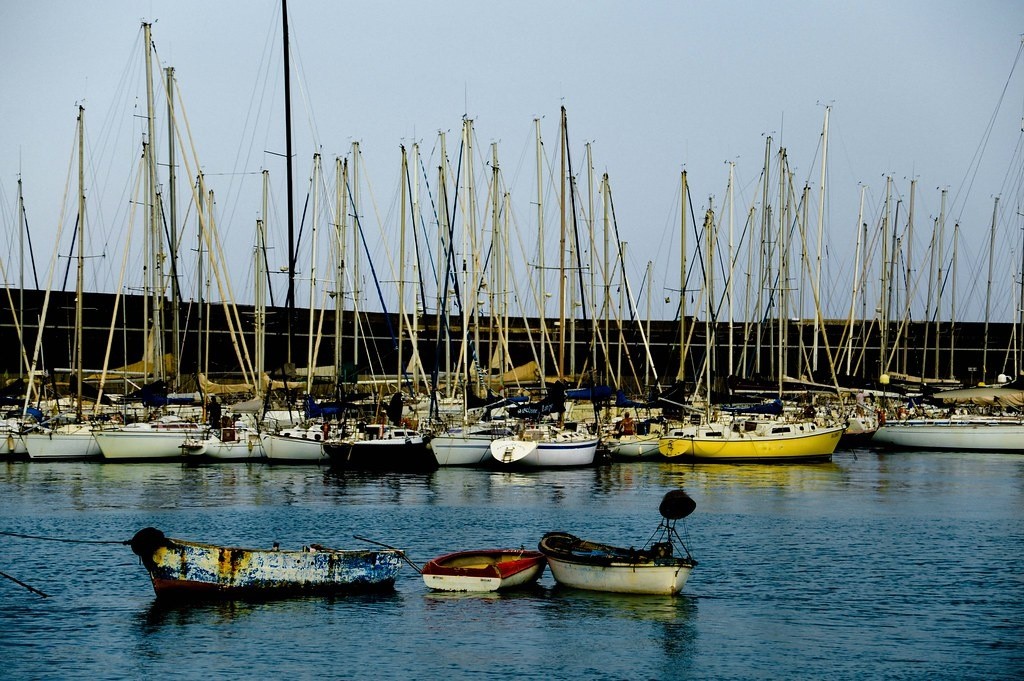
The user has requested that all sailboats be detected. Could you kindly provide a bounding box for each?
[0,0,1024,473]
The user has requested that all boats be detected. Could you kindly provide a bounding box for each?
[131,523,407,605]
[420,547,549,594]
[535,489,704,597]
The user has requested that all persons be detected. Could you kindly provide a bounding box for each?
[855,389,866,419]
[803,398,817,418]
[616,412,636,439]
[206,397,221,429]
